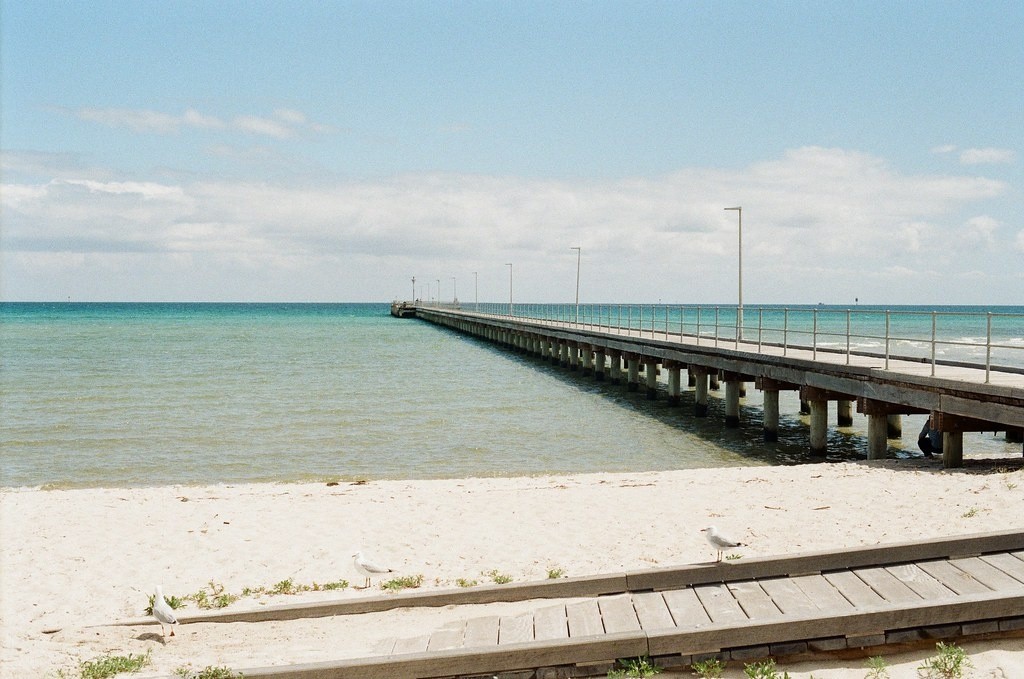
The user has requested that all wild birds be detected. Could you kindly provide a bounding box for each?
[153,584,181,638]
[701,526,750,564]
[351,551,400,589]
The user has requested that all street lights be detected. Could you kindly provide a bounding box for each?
[471,272,478,311]
[570,247,580,321]
[452,277,455,306]
[436,279,440,307]
[723,206,745,342]
[505,263,513,315]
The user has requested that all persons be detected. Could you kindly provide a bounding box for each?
[918,415,943,459]
[416,298,418,301]
